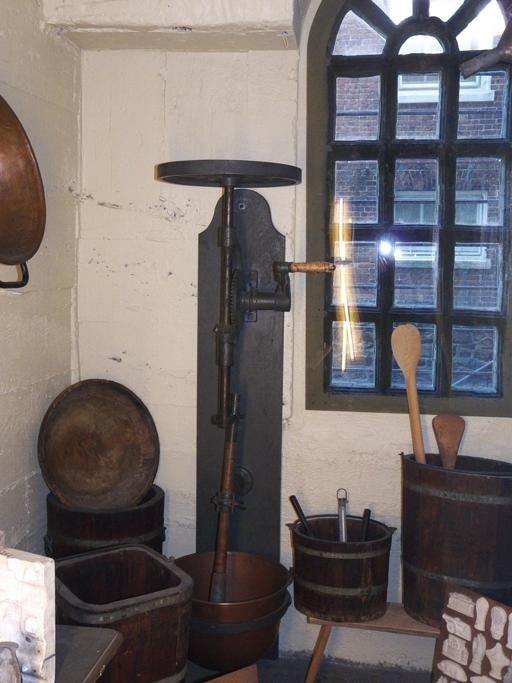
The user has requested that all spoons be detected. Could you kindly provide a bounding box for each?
[391,324,426,465]
[436,414,463,471]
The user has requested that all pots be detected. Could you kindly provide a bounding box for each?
[0,94,46,288]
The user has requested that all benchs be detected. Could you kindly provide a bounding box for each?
[305,601,443,683]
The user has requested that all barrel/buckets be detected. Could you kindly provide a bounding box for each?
[44,485,167,559]
[174,551,293,670]
[290,513,391,621]
[402,453,511,630]
[54,544,194,683]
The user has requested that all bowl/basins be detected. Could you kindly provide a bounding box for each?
[37,377,160,511]
